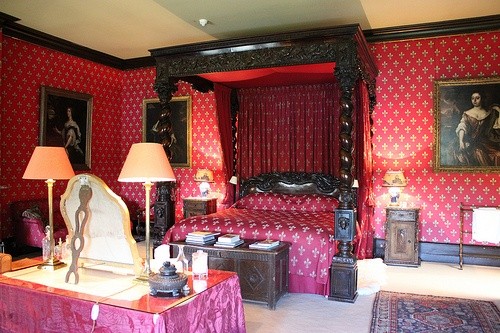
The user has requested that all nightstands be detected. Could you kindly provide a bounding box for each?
[183,197,218,218]
[383,207,421,267]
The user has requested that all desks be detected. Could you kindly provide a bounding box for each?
[0,256,246,333]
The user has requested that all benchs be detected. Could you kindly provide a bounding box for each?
[7,198,134,256]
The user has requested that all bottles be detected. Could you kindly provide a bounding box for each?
[62,235,69,258]
[42,226,55,261]
[55,242,62,256]
[175,245,188,274]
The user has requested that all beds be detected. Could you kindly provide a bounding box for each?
[148,23,379,303]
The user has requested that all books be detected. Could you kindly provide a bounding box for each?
[184,229,280,252]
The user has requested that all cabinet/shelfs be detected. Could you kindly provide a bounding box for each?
[169,231,292,311]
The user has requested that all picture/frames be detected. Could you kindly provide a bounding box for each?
[38,85,94,170]
[431,75,500,173]
[142,96,192,168]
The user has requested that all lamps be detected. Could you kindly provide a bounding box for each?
[194,169,215,198]
[382,171,407,206]
[21,147,75,270]
[118,143,176,281]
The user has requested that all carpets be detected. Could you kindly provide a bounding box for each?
[371,290,500,333]
